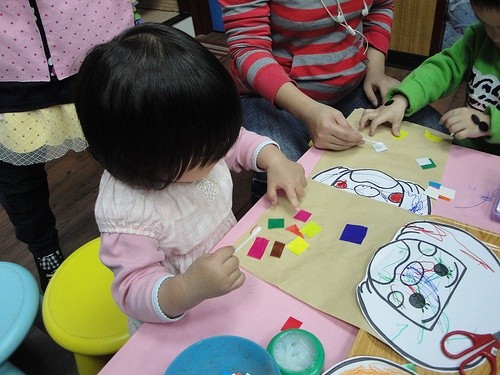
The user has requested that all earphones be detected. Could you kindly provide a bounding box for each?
[362,2,369,16]
[337,11,344,22]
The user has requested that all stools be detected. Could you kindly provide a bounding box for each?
[43,236,130,375]
[0,261,44,375]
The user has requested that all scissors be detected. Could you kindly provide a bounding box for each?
[441,331,500,375]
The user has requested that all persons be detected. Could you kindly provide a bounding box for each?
[358,0,499,155]
[67,21,308,340]
[218,0,450,208]
[0,0,136,293]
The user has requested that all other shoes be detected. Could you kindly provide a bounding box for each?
[34,248,64,292]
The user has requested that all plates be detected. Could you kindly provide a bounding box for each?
[164,334,281,375]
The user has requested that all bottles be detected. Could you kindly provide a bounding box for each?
[266,328,324,375]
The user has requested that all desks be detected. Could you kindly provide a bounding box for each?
[98,108,500,375]
